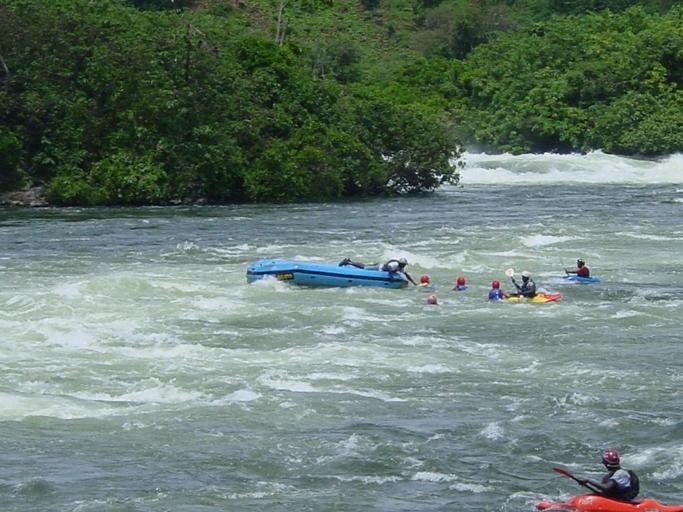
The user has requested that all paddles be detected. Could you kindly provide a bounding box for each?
[553,467,601,493]
[505,268,519,294]
[561,261,570,278]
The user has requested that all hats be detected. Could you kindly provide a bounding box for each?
[399,258,408,265]
[603,450,619,463]
[522,271,530,278]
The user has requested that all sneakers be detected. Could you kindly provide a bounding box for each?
[338,258,351,267]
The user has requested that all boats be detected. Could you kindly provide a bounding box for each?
[502,275,601,304]
[246,259,408,289]
[535,492,683,512]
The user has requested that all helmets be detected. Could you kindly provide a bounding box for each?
[428,295,437,303]
[493,281,499,288]
[458,278,465,285]
[421,275,429,283]
[578,259,585,265]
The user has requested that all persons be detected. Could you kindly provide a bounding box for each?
[337,256,417,286]
[578,448,639,502]
[428,294,437,305]
[566,258,589,278]
[511,270,536,297]
[450,276,469,291]
[416,274,432,287]
[488,280,509,302]
